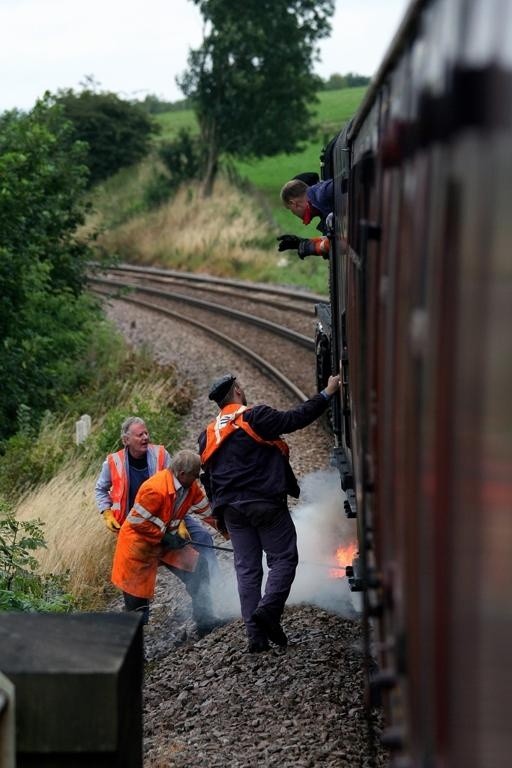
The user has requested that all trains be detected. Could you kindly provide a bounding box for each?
[315,0,512,765]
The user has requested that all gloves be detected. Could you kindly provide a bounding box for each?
[164,520,192,550]
[103,510,120,532]
[276,234,317,260]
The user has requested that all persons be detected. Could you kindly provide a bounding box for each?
[196,374,341,653]
[277,180,336,258]
[95,416,221,590]
[292,171,320,187]
[112,449,231,637]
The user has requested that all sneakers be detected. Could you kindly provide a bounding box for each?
[195,617,234,637]
[250,607,288,652]
[245,634,270,655]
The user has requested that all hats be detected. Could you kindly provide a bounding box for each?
[209,374,235,402]
